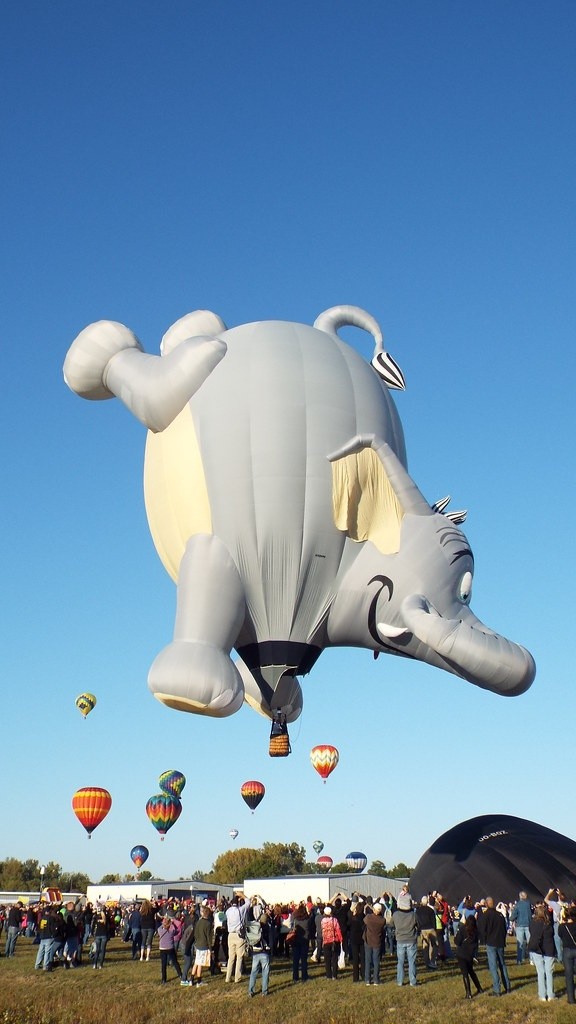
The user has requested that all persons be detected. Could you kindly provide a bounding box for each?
[0,885,576,1004]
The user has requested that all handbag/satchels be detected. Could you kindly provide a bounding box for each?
[239,927,246,939]
[286,925,296,944]
[311,949,319,963]
[337,944,345,970]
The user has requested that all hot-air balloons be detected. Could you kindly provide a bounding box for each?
[71,787,112,840]
[229,829,239,840]
[76,692,97,720]
[345,851,367,873]
[130,844,149,871]
[146,794,183,841]
[157,769,186,800]
[309,744,340,784]
[240,780,265,815]
[316,855,333,874]
[61,302,538,758]
[406,814,576,915]
[313,840,324,858]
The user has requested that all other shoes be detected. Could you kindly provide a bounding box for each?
[261,993,270,998]
[248,992,254,998]
[6,954,15,957]
[365,980,371,986]
[302,978,311,983]
[160,979,167,985]
[397,982,403,987]
[292,980,299,985]
[18,932,36,938]
[409,981,422,986]
[35,956,104,971]
[327,976,339,981]
[373,982,382,987]
[179,979,209,988]
[133,955,151,962]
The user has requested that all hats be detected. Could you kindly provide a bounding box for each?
[17,900,24,908]
[323,907,332,915]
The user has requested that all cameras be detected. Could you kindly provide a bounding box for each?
[554,889,558,891]
